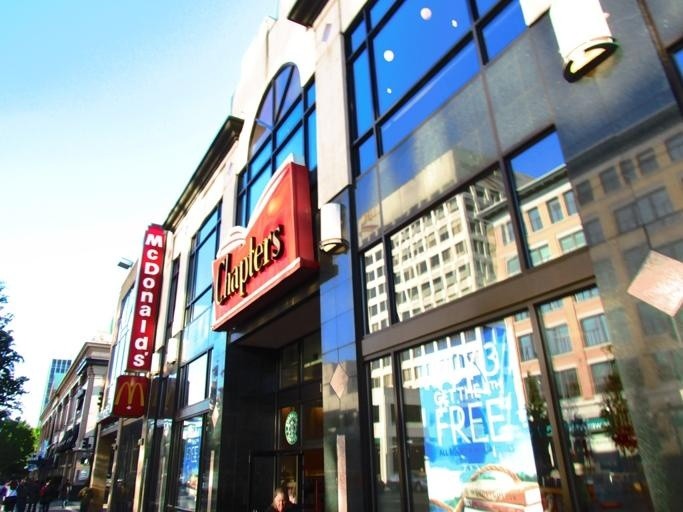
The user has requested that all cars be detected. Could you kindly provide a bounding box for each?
[387,469,430,493]
[374,478,385,494]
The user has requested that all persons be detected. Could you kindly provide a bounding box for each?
[76,486,104,511]
[0,478,54,511]
[266,487,295,511]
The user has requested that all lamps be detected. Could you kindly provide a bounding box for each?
[319,203,349,254]
[550,0,618,82]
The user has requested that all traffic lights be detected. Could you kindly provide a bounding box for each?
[80,457,84,464]
[88,444,92,448]
[81,437,88,448]
